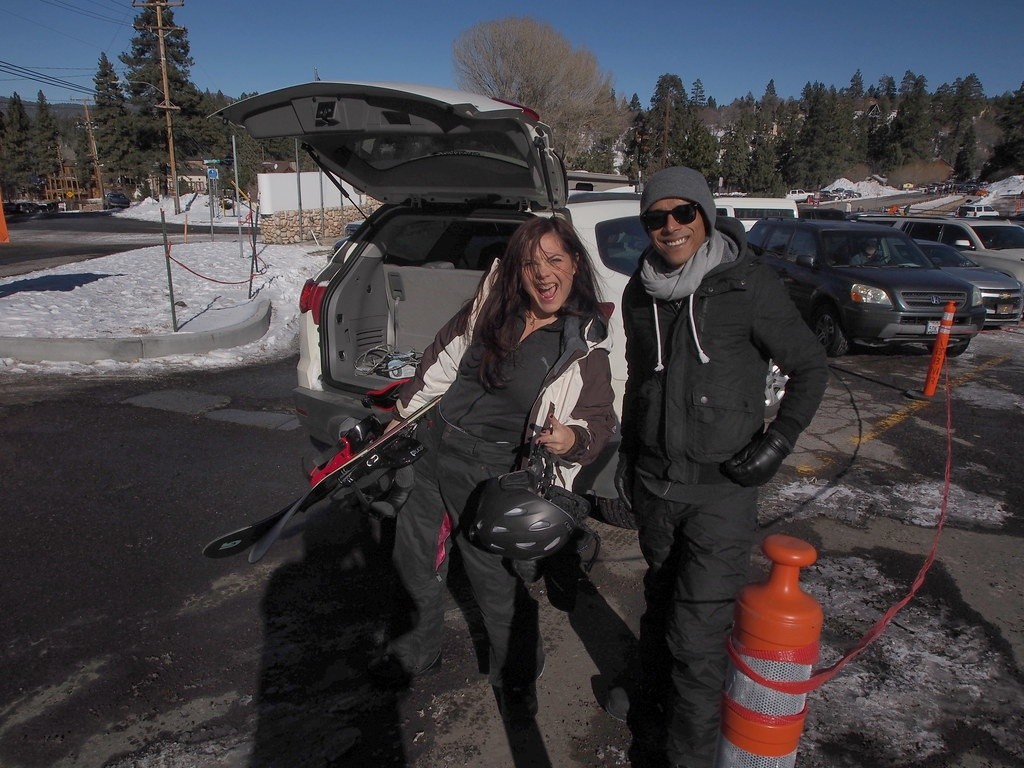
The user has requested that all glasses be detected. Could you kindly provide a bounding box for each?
[642,202,699,230]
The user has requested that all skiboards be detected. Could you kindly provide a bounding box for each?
[201,395,443,564]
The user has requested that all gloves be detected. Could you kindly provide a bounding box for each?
[614,452,637,511]
[722,427,789,488]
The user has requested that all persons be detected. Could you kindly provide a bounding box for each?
[386,216,618,727]
[846,200,853,215]
[858,204,865,212]
[904,204,911,215]
[616,165,828,767]
[851,238,886,266]
[937,181,958,197]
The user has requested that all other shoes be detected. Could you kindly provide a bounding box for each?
[373,650,443,690]
[604,682,667,722]
[500,681,537,719]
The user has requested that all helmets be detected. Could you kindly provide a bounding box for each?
[465,472,579,559]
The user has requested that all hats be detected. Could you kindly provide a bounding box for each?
[640,167,717,237]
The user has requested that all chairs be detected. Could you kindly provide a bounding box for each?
[478,245,507,271]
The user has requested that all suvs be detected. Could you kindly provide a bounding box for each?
[954,203,1000,219]
[203,76,797,530]
[785,189,815,202]
[737,216,987,357]
[854,213,1023,292]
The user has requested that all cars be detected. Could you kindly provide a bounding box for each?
[878,236,1024,326]
[813,187,862,200]
[104,192,130,210]
[3,202,59,216]
